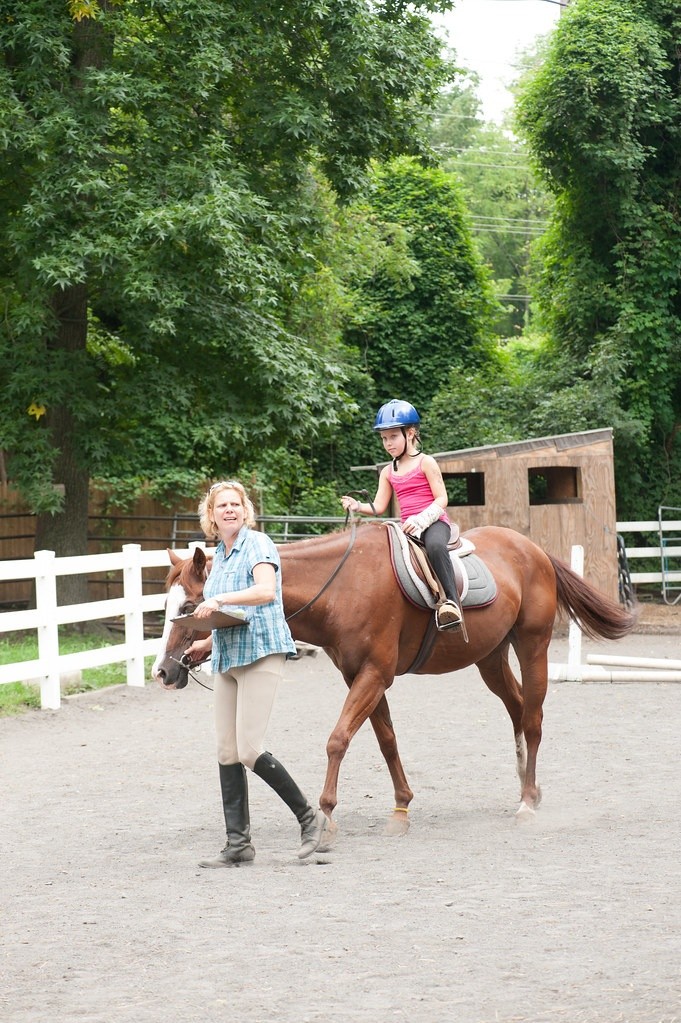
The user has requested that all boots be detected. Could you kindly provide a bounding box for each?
[199,760,254,868]
[251,751,331,858]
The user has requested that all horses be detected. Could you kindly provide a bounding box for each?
[148,521,643,853]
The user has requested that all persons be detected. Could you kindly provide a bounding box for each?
[339,398,463,635]
[184,480,332,868]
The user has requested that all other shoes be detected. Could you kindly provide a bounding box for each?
[438,603,463,633]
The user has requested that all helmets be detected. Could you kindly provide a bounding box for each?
[373,399,420,431]
